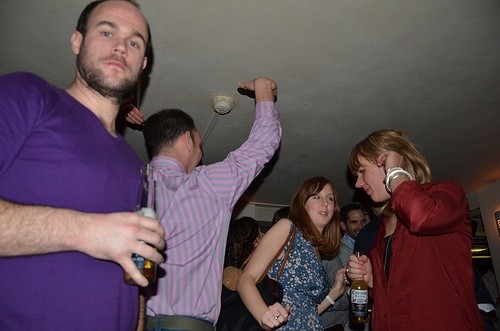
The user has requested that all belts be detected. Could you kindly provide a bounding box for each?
[144,317,216,331]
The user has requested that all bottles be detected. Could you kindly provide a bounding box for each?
[348,254,371,325]
[123,162,158,289]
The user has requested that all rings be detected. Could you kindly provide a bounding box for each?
[274,314,281,320]
[347,269,348,272]
[126,113,129,116]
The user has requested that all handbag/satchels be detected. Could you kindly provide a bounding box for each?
[216,266,282,331]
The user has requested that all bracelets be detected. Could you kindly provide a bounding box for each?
[386,167,412,192]
[326,296,335,305]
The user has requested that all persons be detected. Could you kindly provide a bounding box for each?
[0,0,165,331]
[120,76,282,331]
[237,176,346,331]
[216,195,389,331]
[347,130,484,331]
